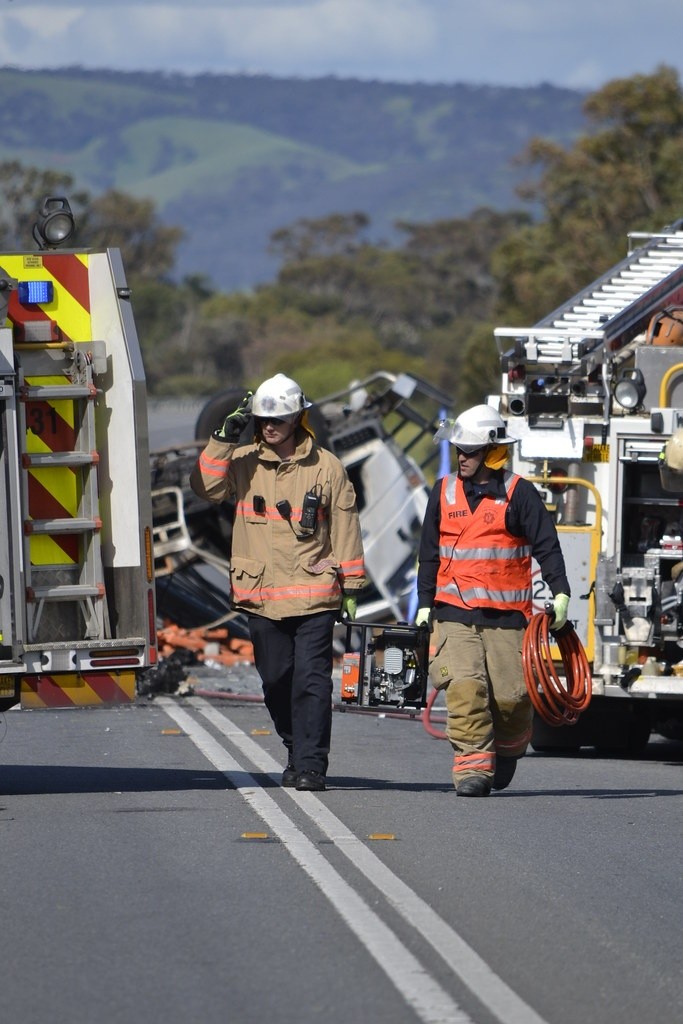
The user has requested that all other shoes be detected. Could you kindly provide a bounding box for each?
[455,774,492,796]
[492,755,518,790]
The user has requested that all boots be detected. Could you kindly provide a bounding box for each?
[295,768,325,792]
[281,743,296,787]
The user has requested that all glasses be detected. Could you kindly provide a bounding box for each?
[260,418,283,428]
[456,447,477,459]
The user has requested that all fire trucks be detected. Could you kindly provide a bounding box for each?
[0,193,164,727]
[486,218,682,751]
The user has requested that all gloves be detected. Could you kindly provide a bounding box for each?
[217,391,252,440]
[414,606,434,633]
[545,593,570,634]
[337,595,356,624]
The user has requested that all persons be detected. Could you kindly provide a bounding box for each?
[192,373,367,791]
[416,405,572,799]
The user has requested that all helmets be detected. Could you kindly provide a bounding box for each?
[252,373,313,418]
[433,404,517,454]
